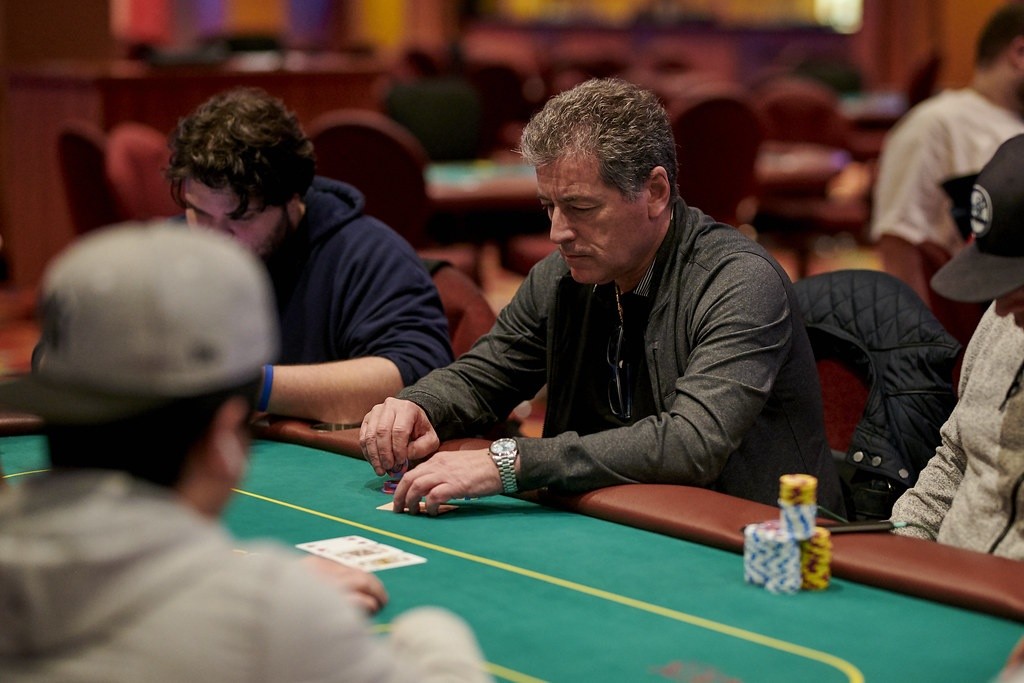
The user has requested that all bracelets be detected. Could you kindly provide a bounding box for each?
[257,364,273,412]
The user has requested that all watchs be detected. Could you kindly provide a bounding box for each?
[487,438,519,494]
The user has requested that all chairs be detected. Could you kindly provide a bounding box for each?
[421,258,495,358]
[873,233,945,306]
[753,94,850,147]
[57,124,115,235]
[307,118,422,244]
[795,264,965,524]
[107,122,177,221]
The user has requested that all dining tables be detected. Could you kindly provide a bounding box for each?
[671,94,762,221]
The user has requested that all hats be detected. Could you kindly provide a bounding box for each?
[34,220,277,395]
[930,134,1024,301]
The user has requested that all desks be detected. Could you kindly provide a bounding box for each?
[426,154,843,277]
[0,403,1024,683]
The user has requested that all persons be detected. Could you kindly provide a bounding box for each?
[0,220,492,683]
[359,77,847,522]
[869,0,1024,241]
[161,85,455,424]
[889,134,1024,561]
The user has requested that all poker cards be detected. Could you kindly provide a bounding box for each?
[295,535,427,574]
[378,502,461,515]
